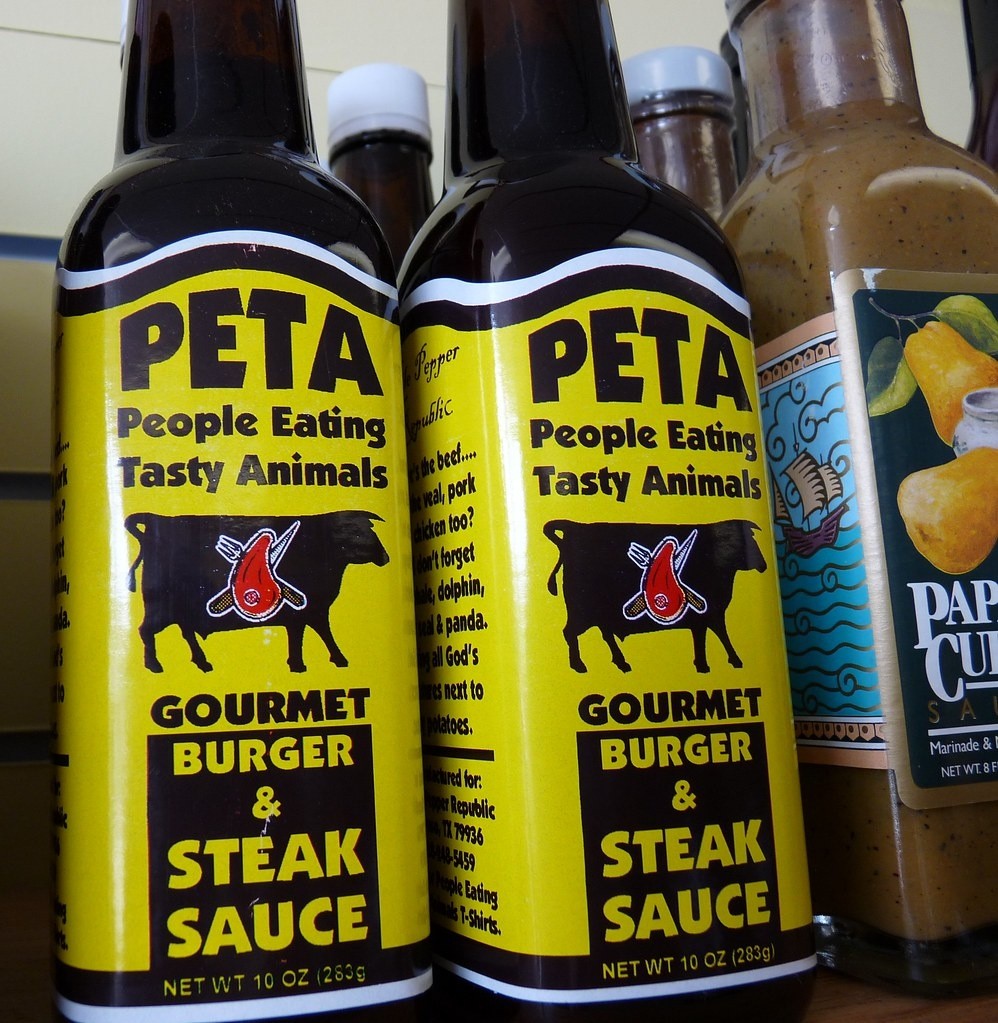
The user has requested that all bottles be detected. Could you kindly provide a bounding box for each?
[616,0,998,999]
[50,1,441,1023]
[321,62,435,275]
[398,0,811,1023]
